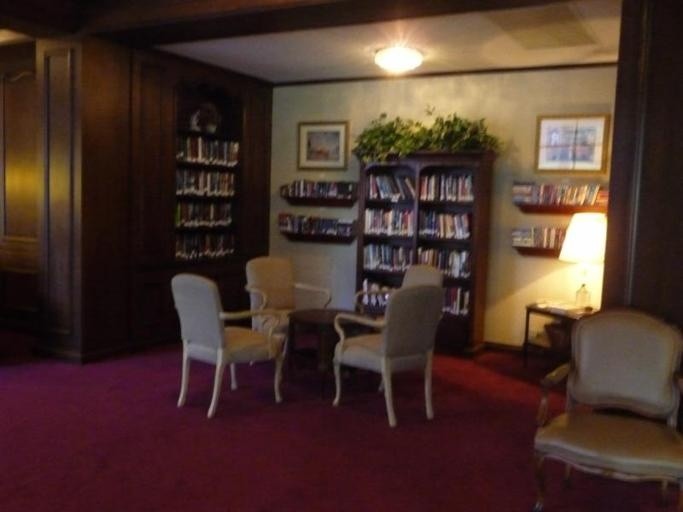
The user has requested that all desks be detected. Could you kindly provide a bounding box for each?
[521,301,590,375]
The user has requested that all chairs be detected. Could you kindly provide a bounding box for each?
[525,307,683,510]
[169,254,332,419]
[332,261,446,428]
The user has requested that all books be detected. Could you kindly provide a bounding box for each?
[277,174,473,317]
[173,136,239,260]
[511,180,609,250]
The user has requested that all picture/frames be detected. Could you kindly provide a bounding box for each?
[531,112,614,176]
[295,117,350,172]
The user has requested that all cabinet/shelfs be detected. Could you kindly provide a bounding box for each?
[282,190,356,245]
[351,149,497,357]
[512,201,610,260]
[130,47,275,350]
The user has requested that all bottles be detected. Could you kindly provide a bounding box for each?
[574,284,591,307]
